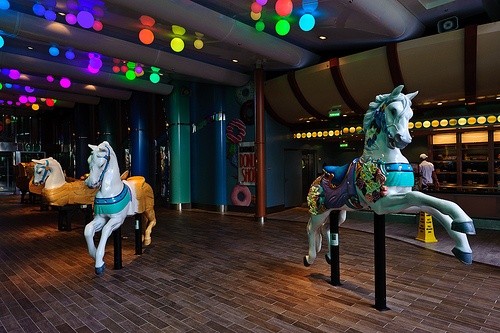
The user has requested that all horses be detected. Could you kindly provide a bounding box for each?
[31,157,129,210]
[302,85,477,269]
[82,141,156,273]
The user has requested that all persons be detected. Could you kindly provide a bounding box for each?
[418,154,439,190]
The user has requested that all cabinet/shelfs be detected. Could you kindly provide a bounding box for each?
[434,159,500,190]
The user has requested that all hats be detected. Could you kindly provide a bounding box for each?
[420,154,428,159]
